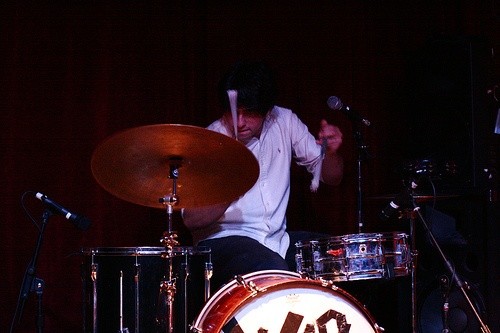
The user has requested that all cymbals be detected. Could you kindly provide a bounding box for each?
[91,124,261,209]
[369,192,461,203]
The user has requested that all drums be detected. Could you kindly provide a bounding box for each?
[296,232,413,282]
[81,246,213,333]
[190,268,382,333]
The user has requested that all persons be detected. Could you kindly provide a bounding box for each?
[180,72,345,285]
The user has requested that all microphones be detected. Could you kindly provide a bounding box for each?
[35,192,90,230]
[327,96,371,126]
[378,166,426,220]
